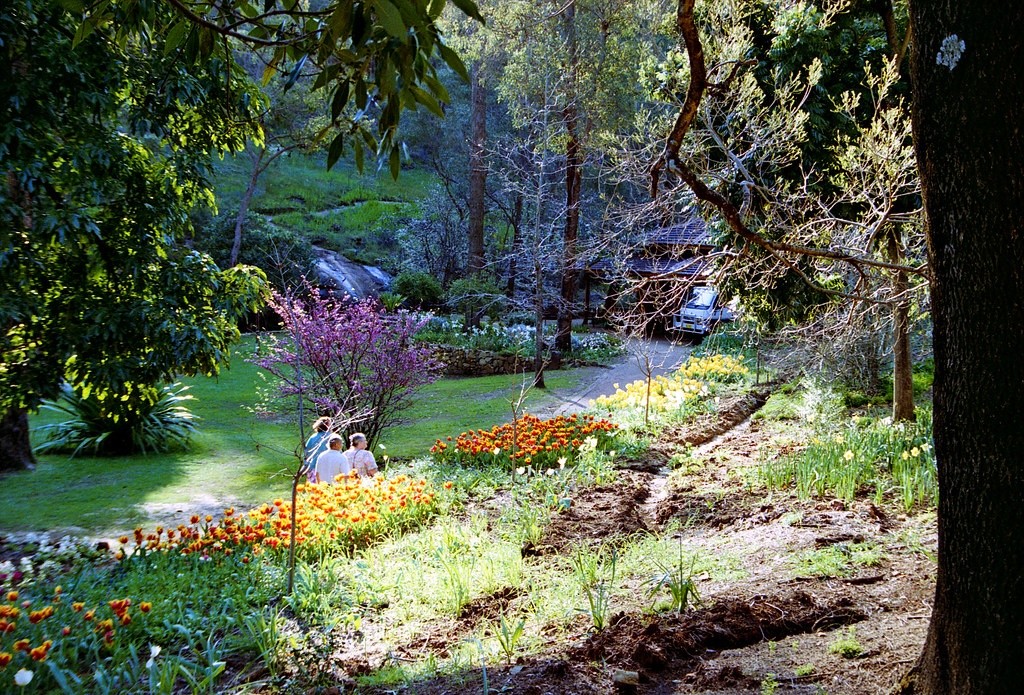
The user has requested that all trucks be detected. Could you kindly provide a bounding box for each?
[673,285,740,345]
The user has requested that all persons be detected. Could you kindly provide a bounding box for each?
[305,416,335,473]
[342,432,378,478]
[315,434,350,483]
